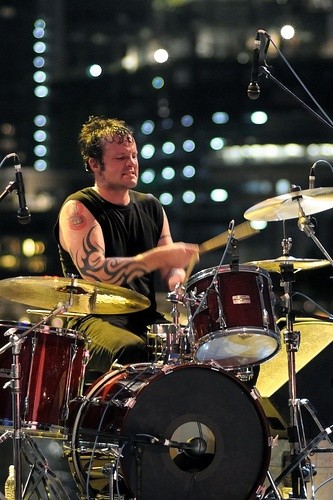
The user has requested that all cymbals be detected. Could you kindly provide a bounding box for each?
[0,276,151,315]
[243,186,333,223]
[240,255,333,272]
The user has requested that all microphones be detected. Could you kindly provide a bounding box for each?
[160,437,207,458]
[226,223,235,255]
[273,292,297,305]
[308,166,316,220]
[14,155,31,224]
[248,31,261,99]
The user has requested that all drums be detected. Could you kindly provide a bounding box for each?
[63,362,272,500]
[0,319,90,441]
[146,324,192,364]
[182,263,282,370]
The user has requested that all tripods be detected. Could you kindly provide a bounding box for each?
[0,292,75,500]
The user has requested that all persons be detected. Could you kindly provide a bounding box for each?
[53,116,200,371]
